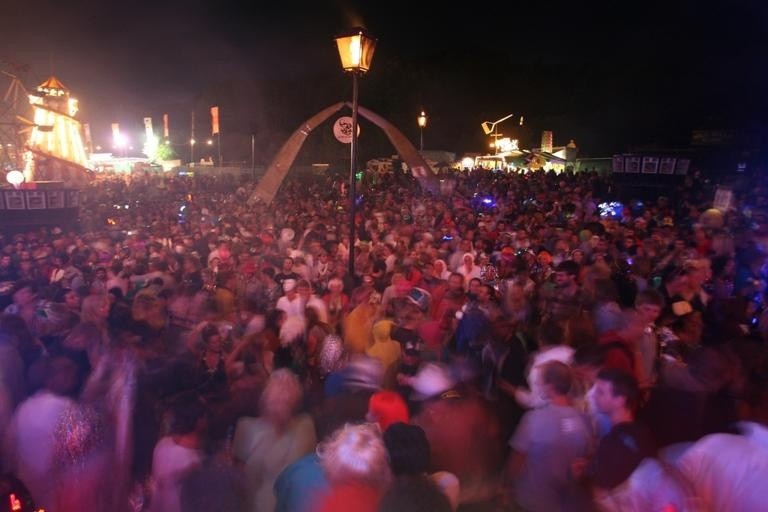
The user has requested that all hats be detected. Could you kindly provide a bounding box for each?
[401,338,421,357]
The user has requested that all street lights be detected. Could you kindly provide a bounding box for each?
[417,108,428,153]
[334,20,379,280]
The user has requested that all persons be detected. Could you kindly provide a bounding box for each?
[0,162,767,510]
